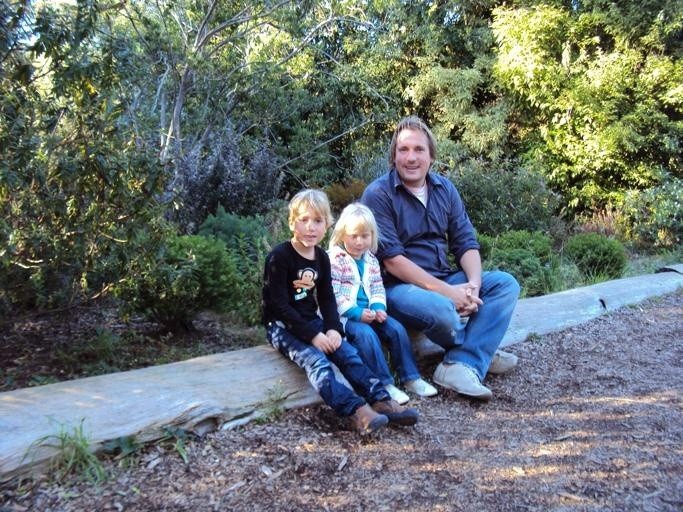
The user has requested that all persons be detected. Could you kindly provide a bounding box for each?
[314,200,438,404]
[359,116,519,402]
[261,189,421,439]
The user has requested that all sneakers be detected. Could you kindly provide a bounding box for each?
[490,350,518,373]
[433,363,493,403]
[372,399,419,425]
[385,383,409,405]
[405,379,436,396]
[352,405,388,435]
